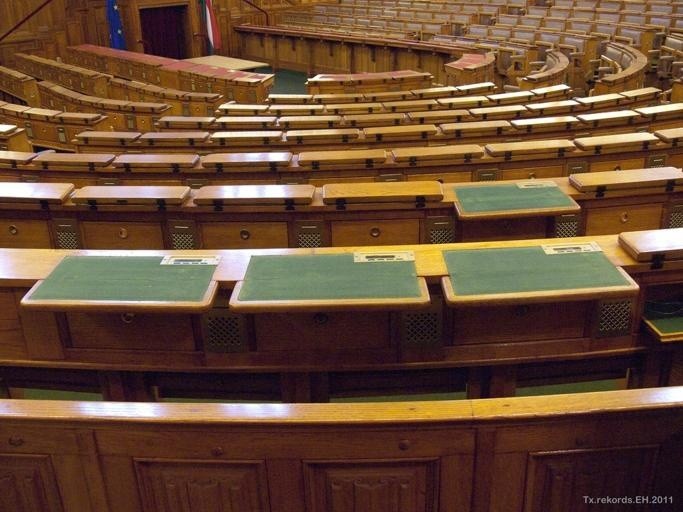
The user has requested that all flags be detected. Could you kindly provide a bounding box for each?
[106,1,127,50]
[200,0,223,56]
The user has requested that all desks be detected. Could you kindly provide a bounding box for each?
[0,0,683,512]
